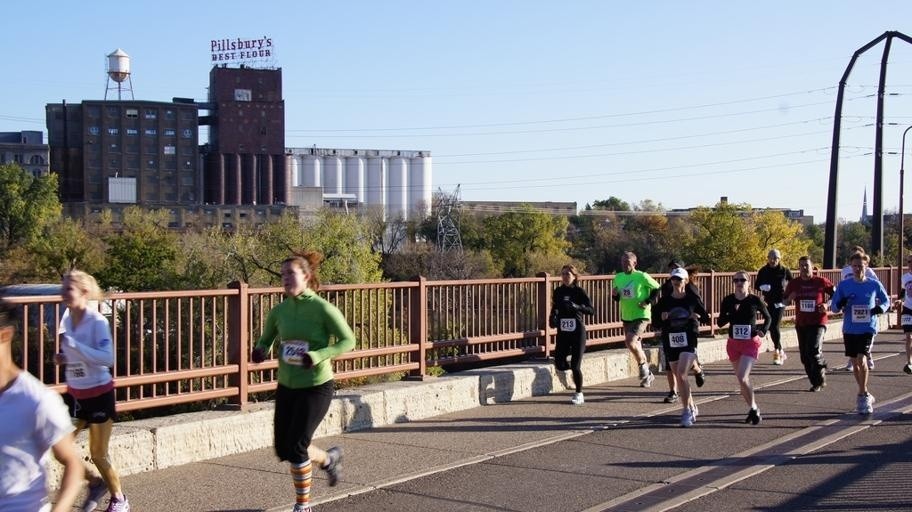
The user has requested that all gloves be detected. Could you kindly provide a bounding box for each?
[300,351,315,371]
[567,300,580,311]
[761,284,772,292]
[251,346,268,364]
[549,314,560,328]
[869,306,883,319]
[836,293,856,312]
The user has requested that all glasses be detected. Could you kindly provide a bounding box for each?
[732,277,748,282]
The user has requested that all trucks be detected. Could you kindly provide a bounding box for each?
[0,284,122,362]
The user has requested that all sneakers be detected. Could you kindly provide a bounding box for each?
[679,404,699,428]
[773,352,787,366]
[810,381,826,394]
[903,363,912,375]
[571,391,585,405]
[846,361,855,374]
[320,446,346,488]
[856,391,876,415]
[744,407,762,426]
[664,390,680,404]
[867,359,875,371]
[695,364,707,388]
[108,495,131,512]
[638,362,656,388]
[83,478,108,512]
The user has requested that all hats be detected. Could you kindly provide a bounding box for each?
[767,247,783,264]
[670,267,688,282]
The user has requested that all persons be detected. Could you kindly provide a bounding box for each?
[831,252,890,414]
[54,271,131,512]
[549,265,594,404]
[754,250,795,365]
[661,259,706,403]
[652,268,708,427]
[782,256,836,392]
[612,252,661,387]
[841,246,879,372]
[252,253,356,512]
[0,299,85,512]
[717,271,772,424]
[895,255,912,374]
